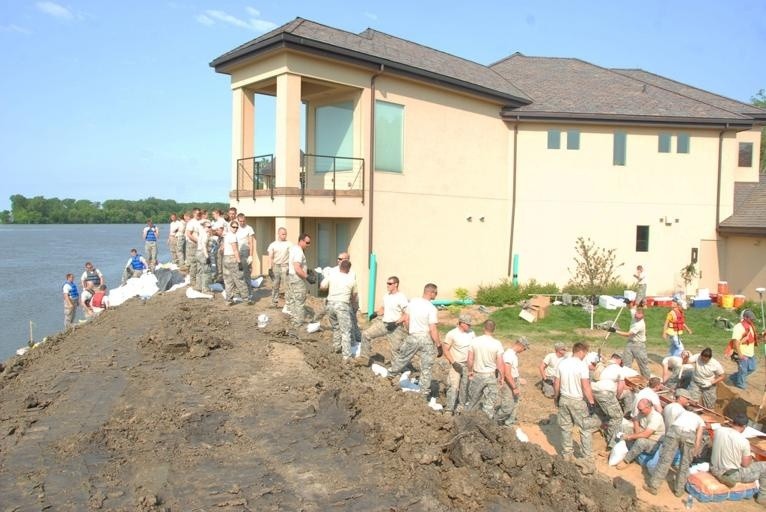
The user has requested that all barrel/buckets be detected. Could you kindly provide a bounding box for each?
[734,295,746,307]
[710,292,717,303]
[716,293,721,305]
[718,281,730,294]
[721,295,734,308]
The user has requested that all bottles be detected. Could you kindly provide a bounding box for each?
[687,493,693,510]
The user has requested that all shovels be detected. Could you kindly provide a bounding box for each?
[746,386,765,431]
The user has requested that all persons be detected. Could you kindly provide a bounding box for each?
[121,221,159,288]
[166,206,254,306]
[631,264,649,310]
[62,262,110,330]
[318,253,363,363]
[540,340,766,511]
[267,227,316,327]
[662,300,693,370]
[725,309,760,390]
[607,297,651,380]
[359,276,444,403]
[445,316,528,427]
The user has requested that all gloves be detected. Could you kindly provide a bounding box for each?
[452,361,463,373]
[608,327,615,332]
[306,275,315,284]
[387,324,396,331]
[307,268,314,276]
[269,269,274,281]
[238,263,242,270]
[246,256,252,265]
[436,344,442,357]
[623,298,632,304]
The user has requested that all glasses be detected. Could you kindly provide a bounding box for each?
[387,282,396,285]
[231,226,237,229]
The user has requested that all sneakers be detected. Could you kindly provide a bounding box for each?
[674,489,684,497]
[643,483,658,496]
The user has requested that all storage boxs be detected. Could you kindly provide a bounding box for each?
[597,280,746,310]
[518,296,552,324]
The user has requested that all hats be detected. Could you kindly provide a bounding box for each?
[459,314,472,325]
[554,342,567,352]
[743,310,757,320]
[611,351,623,358]
[675,388,691,399]
[677,299,687,310]
[517,336,530,350]
